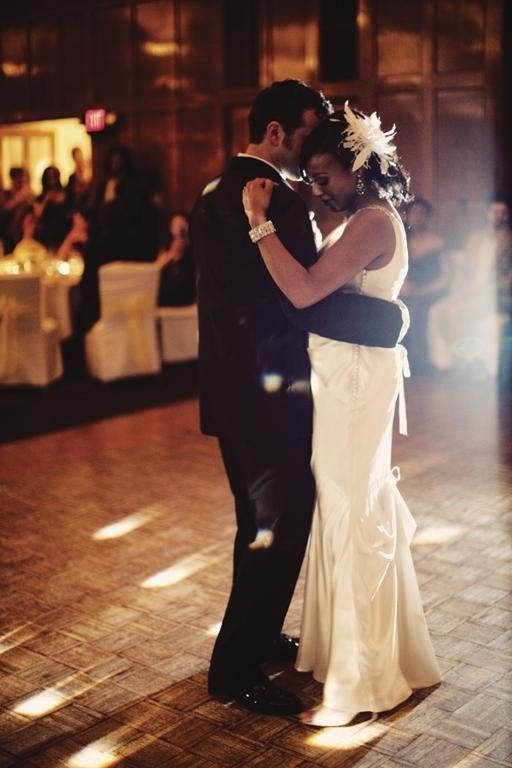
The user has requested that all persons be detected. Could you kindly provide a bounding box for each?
[241,106,446,729]
[424,190,511,375]
[194,77,409,718]
[1,141,194,309]
[401,196,456,370]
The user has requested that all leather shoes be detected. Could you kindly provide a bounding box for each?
[231,683,301,716]
[272,634,300,662]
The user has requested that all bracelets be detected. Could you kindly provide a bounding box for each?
[246,218,276,245]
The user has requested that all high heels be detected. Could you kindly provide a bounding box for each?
[297,703,378,726]
